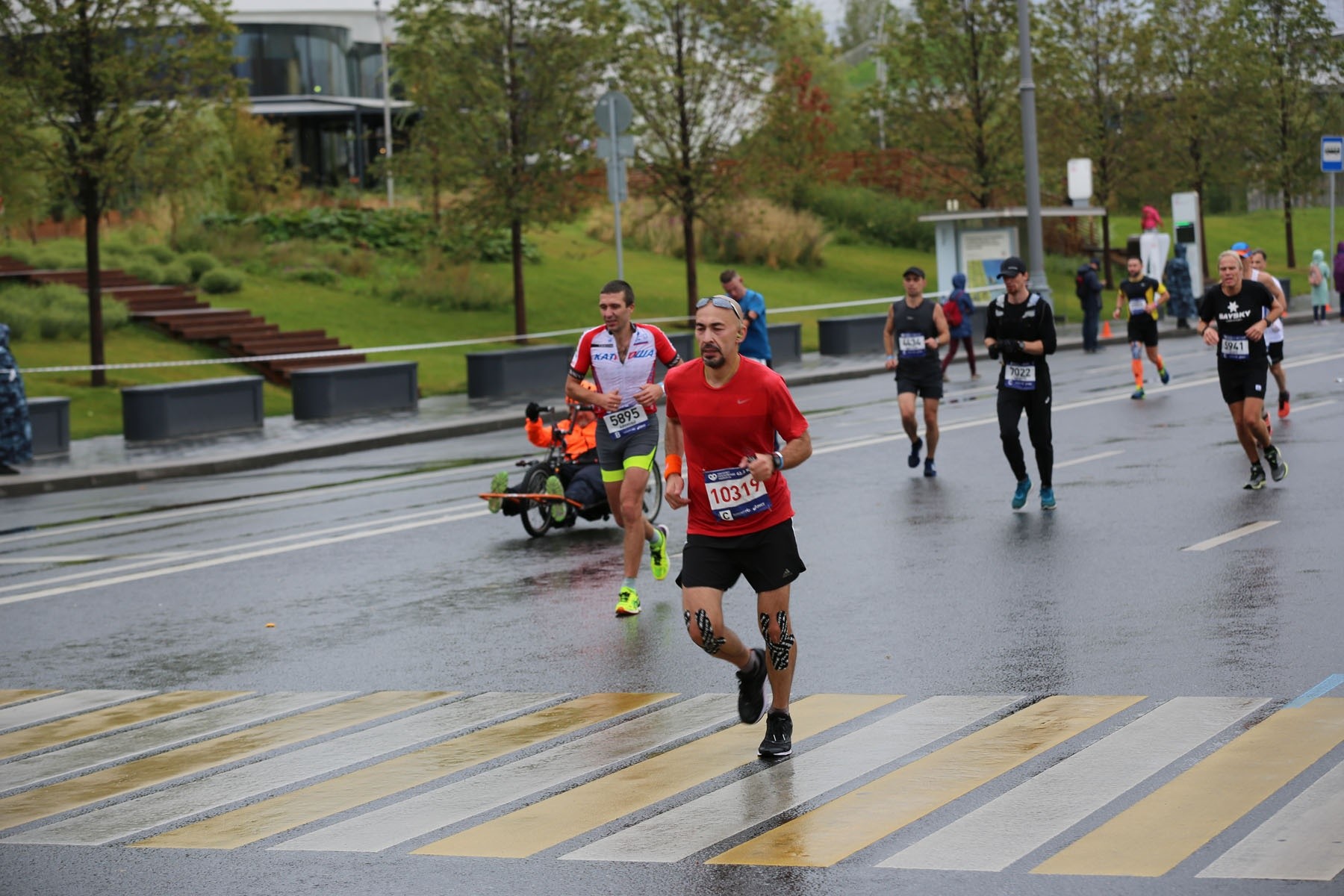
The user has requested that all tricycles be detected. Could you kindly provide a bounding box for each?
[478,401,663,538]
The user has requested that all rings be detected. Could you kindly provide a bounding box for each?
[614,404,617,408]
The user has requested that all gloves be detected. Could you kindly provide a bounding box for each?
[526,402,540,422]
[1003,338,1024,354]
[988,342,1000,360]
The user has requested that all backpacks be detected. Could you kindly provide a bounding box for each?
[1075,270,1092,298]
[1307,262,1324,287]
[942,291,969,327]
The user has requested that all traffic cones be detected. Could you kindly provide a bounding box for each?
[1099,319,1114,338]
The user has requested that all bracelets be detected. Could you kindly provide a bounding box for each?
[935,338,940,345]
[775,451,784,471]
[1200,325,1208,337]
[885,355,894,359]
[1116,307,1122,309]
[663,455,682,480]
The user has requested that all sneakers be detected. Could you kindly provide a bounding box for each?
[736,647,769,725]
[615,586,642,614]
[1263,444,1288,482]
[757,710,793,757]
[1243,466,1267,490]
[1038,487,1057,511]
[1011,473,1032,509]
[649,524,671,580]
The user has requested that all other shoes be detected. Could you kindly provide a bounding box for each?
[924,457,937,477]
[1176,322,1190,330]
[545,473,573,523]
[0,467,19,475]
[488,470,508,513]
[1132,390,1144,399]
[908,437,923,468]
[1340,316,1344,322]
[971,372,981,378]
[1157,363,1169,384]
[1084,345,1105,354]
[942,378,949,383]
[1314,319,1329,326]
[1278,391,1290,418]
[1257,412,1272,449]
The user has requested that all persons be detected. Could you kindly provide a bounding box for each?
[1076,258,1106,354]
[1197,250,1284,490]
[1218,243,1290,449]
[940,273,983,379]
[1308,249,1331,325]
[1334,241,1344,324]
[883,266,951,478]
[719,270,782,453]
[985,257,1057,510]
[565,280,684,616]
[1113,256,1170,398]
[665,295,812,759]
[1249,249,1284,294]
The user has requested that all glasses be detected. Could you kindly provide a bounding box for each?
[906,277,919,282]
[696,296,743,324]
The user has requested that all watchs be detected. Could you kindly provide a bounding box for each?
[1018,340,1024,350]
[1264,317,1271,327]
[769,452,781,469]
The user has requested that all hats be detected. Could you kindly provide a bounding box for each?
[1090,258,1101,271]
[903,267,924,277]
[1231,241,1253,258]
[996,257,1027,280]
